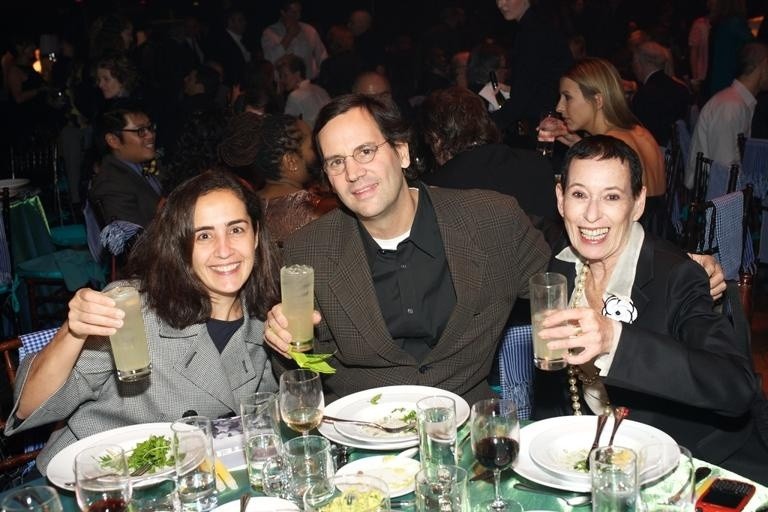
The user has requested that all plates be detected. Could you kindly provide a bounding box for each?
[204,496,303,512]
[75,428,203,482]
[335,392,450,442]
[46,422,209,490]
[334,455,422,496]
[529,416,627,480]
[507,417,626,495]
[315,384,471,449]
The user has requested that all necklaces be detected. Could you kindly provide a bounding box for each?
[566,258,600,417]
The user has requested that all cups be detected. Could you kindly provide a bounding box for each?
[244,391,281,460]
[170,416,218,512]
[100,281,153,382]
[284,436,334,510]
[529,273,568,371]
[278,369,328,476]
[471,398,523,511]
[590,446,639,512]
[415,466,466,512]
[75,445,133,512]
[4,488,63,512]
[247,434,280,500]
[417,398,456,485]
[281,264,316,352]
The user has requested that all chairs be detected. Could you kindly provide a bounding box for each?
[700,182,753,284]
[2,188,96,328]
[495,325,533,423]
[690,151,739,199]
[737,132,767,182]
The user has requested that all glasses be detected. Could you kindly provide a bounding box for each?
[118,123,157,137]
[320,140,388,177]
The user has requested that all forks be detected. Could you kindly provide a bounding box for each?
[320,415,414,434]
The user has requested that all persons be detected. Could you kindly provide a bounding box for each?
[420,85,565,247]
[217,113,338,248]
[259,0,328,92]
[531,132,768,487]
[5,168,281,481]
[85,98,180,232]
[682,41,767,202]
[492,0,571,126]
[535,56,668,197]
[278,53,331,131]
[688,0,722,91]
[263,94,728,412]
[322,0,509,93]
[0,1,270,129]
[629,41,688,133]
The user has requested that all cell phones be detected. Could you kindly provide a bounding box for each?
[695,478,756,512]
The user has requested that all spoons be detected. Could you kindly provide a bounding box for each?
[512,484,587,505]
